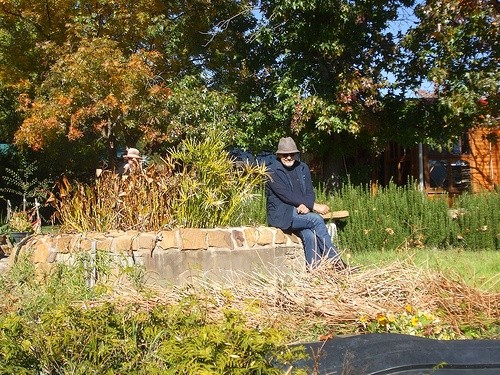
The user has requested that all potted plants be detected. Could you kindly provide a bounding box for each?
[8,210,32,243]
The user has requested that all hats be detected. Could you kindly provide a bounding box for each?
[275,137,300,153]
[122,148,142,158]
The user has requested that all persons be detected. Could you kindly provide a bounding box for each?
[264,136,362,279]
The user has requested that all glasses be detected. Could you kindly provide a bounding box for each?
[128,158,133,160]
[278,154,295,156]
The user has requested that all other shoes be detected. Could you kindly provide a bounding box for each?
[332,261,355,273]
